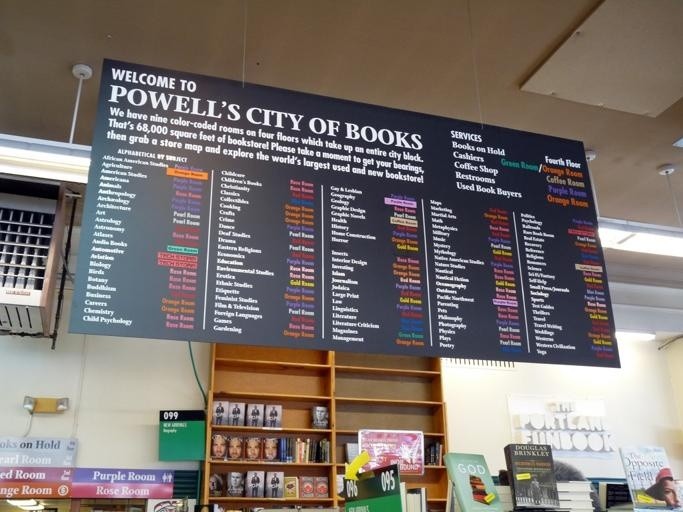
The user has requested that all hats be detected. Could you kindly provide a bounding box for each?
[645,469,672,501]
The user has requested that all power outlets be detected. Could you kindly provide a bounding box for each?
[24,398,66,413]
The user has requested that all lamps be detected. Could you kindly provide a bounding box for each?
[0,63,94,184]
[584,150,683,258]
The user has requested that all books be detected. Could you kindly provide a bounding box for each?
[344,442,359,464]
[209,399,332,512]
[399,440,682,511]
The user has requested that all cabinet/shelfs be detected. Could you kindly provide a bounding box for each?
[200,343,452,512]
